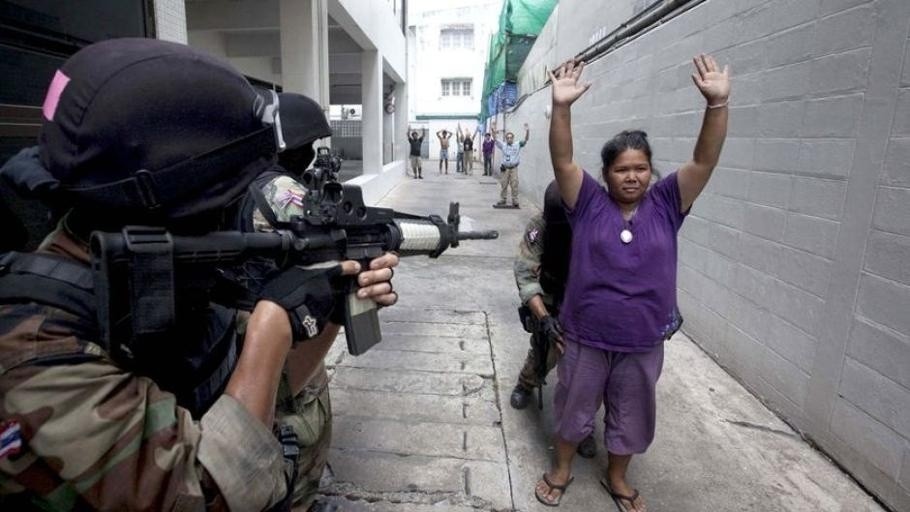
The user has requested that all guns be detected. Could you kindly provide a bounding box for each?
[303,145,342,189]
[533,292,555,409]
[89,180,499,355]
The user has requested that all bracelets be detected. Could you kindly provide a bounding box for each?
[707,95,731,110]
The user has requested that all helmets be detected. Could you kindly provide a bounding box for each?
[275,93,333,149]
[1,38,278,234]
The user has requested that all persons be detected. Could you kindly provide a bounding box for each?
[456,130,466,173]
[458,123,480,177]
[436,129,453,175]
[509,178,598,458]
[535,51,732,512]
[490,122,530,208]
[478,128,495,177]
[224,91,338,510]
[407,124,426,180]
[1,35,402,510]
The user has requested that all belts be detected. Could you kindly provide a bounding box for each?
[505,164,518,169]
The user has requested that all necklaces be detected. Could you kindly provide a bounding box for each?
[620,203,637,243]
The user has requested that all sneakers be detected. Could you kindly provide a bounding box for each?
[576,436,596,458]
[511,385,531,408]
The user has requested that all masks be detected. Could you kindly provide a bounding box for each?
[279,149,314,177]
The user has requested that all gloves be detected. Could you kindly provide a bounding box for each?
[540,314,561,348]
[255,261,341,347]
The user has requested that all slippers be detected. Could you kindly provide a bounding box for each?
[601,476,647,512]
[535,473,574,506]
